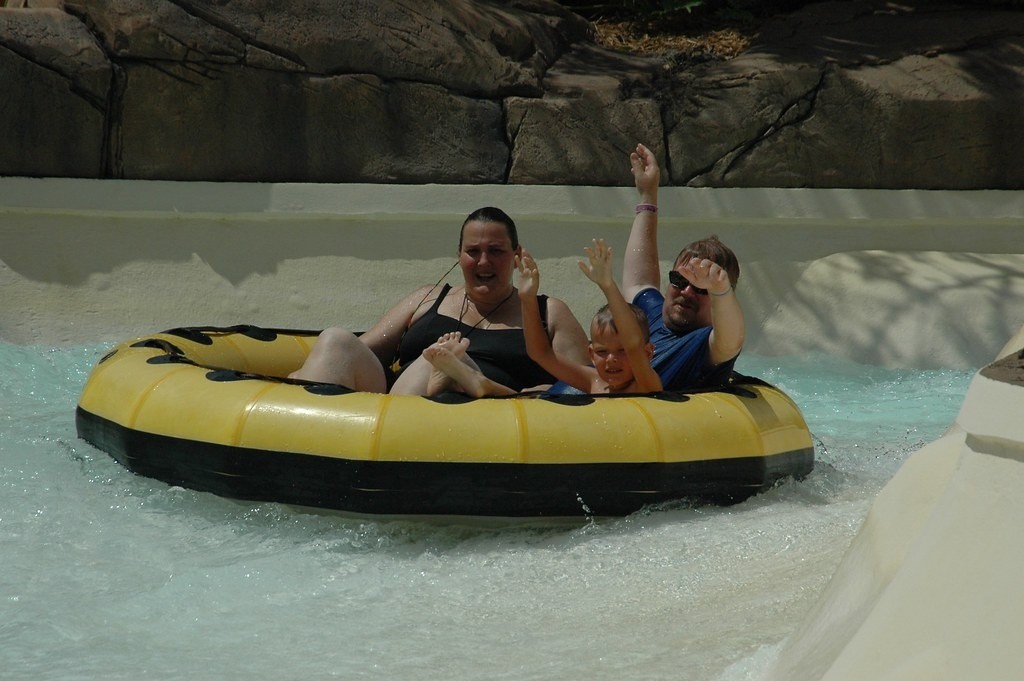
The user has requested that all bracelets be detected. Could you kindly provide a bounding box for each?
[709,286,731,296]
[635,204,657,213]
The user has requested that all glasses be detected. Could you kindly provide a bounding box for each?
[669,271,709,296]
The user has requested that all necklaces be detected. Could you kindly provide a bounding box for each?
[457,287,513,337]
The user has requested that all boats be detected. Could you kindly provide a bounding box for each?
[76,324,814,516]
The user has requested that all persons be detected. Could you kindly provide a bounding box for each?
[422,239,662,397]
[547,144,745,395]
[287,206,590,396]
